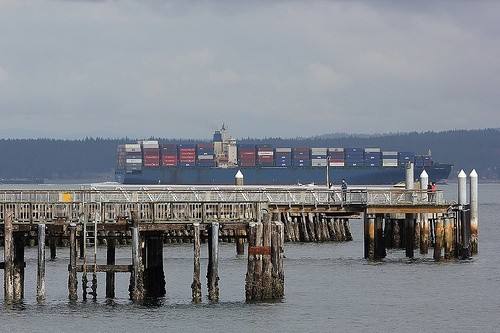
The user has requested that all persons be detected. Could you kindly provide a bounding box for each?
[430,181,436,202]
[342,178,347,201]
[427,180,433,201]
[326,182,335,204]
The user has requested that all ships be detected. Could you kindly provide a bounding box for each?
[116,125,456,189]
